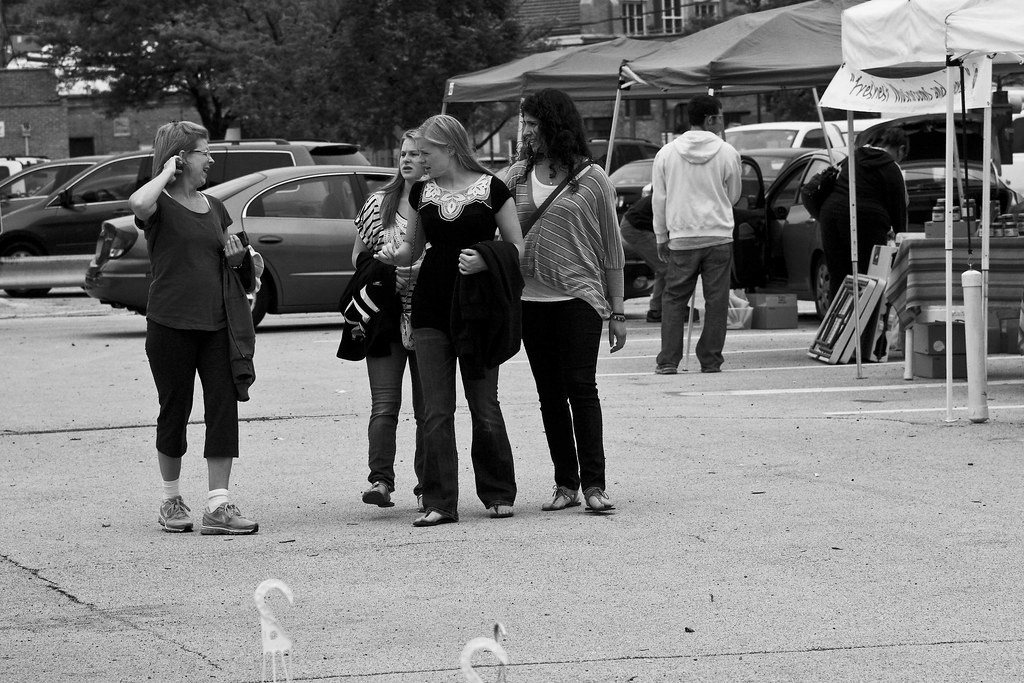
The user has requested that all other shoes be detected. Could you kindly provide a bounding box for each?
[583,487,616,510]
[417,494,425,511]
[647,311,662,322]
[362,481,394,507]
[542,485,581,510]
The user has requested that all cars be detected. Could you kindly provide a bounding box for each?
[0,155,117,216]
[477,157,510,176]
[608,158,656,302]
[828,119,893,157]
[81,166,399,326]
[724,121,847,197]
[731,113,1013,320]
[733,148,821,242]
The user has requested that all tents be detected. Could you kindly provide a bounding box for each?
[440,1,1024,422]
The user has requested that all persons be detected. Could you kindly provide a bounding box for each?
[351,130,425,509]
[494,89,628,511]
[620,194,699,323]
[373,114,525,527]
[819,128,909,308]
[654,96,742,375]
[128,121,258,535]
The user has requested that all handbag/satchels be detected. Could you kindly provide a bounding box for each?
[802,158,846,223]
[401,313,415,350]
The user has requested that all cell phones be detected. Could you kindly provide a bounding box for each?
[235,231,249,247]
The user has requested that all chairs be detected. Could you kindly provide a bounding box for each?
[321,193,350,219]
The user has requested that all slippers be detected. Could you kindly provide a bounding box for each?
[490,505,514,517]
[412,510,457,525]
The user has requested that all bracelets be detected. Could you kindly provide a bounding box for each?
[230,264,242,269]
[611,311,625,322]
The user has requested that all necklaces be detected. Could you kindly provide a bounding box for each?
[550,181,558,185]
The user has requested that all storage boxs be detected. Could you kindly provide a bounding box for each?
[910,353,967,379]
[748,293,799,330]
[912,321,966,355]
[925,221,975,238]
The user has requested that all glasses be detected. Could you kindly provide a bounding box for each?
[189,150,208,156]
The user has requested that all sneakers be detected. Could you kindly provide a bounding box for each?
[158,496,193,531]
[201,503,258,534]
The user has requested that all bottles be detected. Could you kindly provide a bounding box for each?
[977,212,1024,238]
[931,197,976,223]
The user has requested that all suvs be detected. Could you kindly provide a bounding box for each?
[586,136,662,176]
[0,138,373,299]
